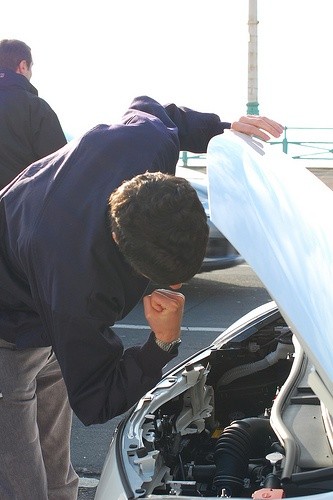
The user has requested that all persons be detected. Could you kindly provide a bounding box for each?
[0,39,68,190]
[0,96,283,500]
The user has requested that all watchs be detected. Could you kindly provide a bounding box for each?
[155,338,181,353]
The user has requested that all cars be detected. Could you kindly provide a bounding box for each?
[175,163,245,274]
[93,128,333,499]
